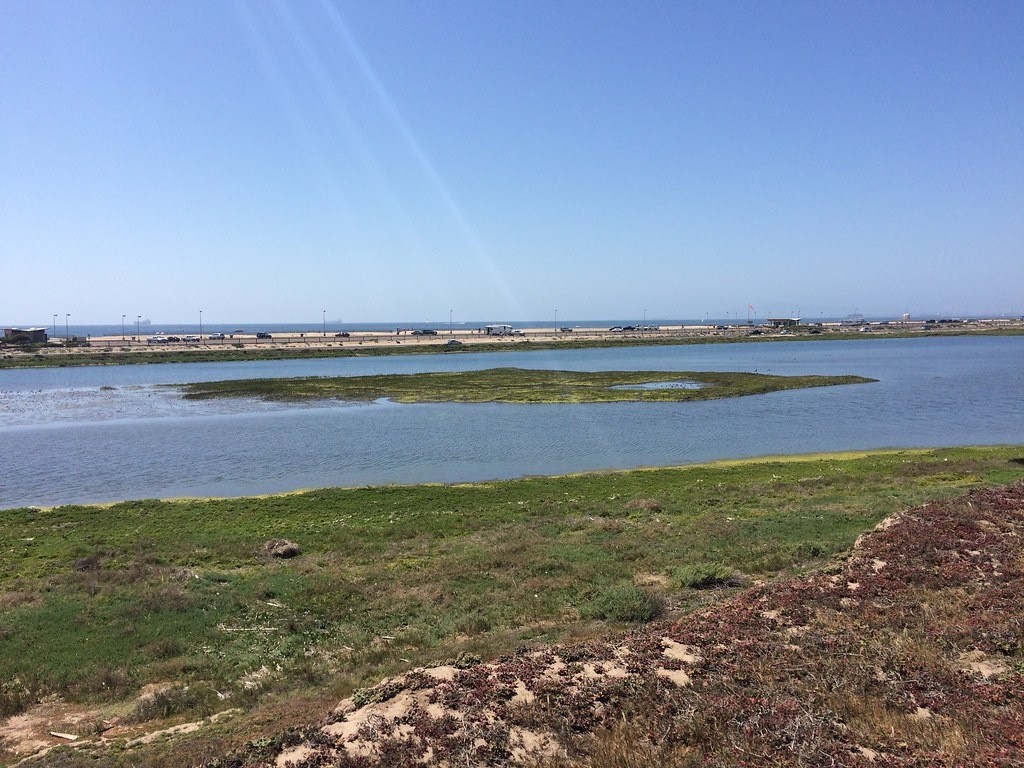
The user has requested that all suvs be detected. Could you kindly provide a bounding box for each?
[256,332,271,339]
[209,332,225,340]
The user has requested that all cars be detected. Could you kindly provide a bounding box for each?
[447,339,462,344]
[608,323,659,333]
[810,329,821,333]
[921,326,930,330]
[560,326,573,333]
[859,327,871,332]
[335,332,350,338]
[781,329,791,334]
[410,329,438,336]
[510,330,525,336]
[749,330,760,335]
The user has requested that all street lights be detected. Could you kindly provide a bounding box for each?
[199,310,202,339]
[323,310,326,337]
[554,309,558,331]
[54,315,58,339]
[137,315,142,341]
[66,313,70,340]
[122,314,126,340]
[449,309,454,336]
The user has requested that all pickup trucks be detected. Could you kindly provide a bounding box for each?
[147,335,200,344]
